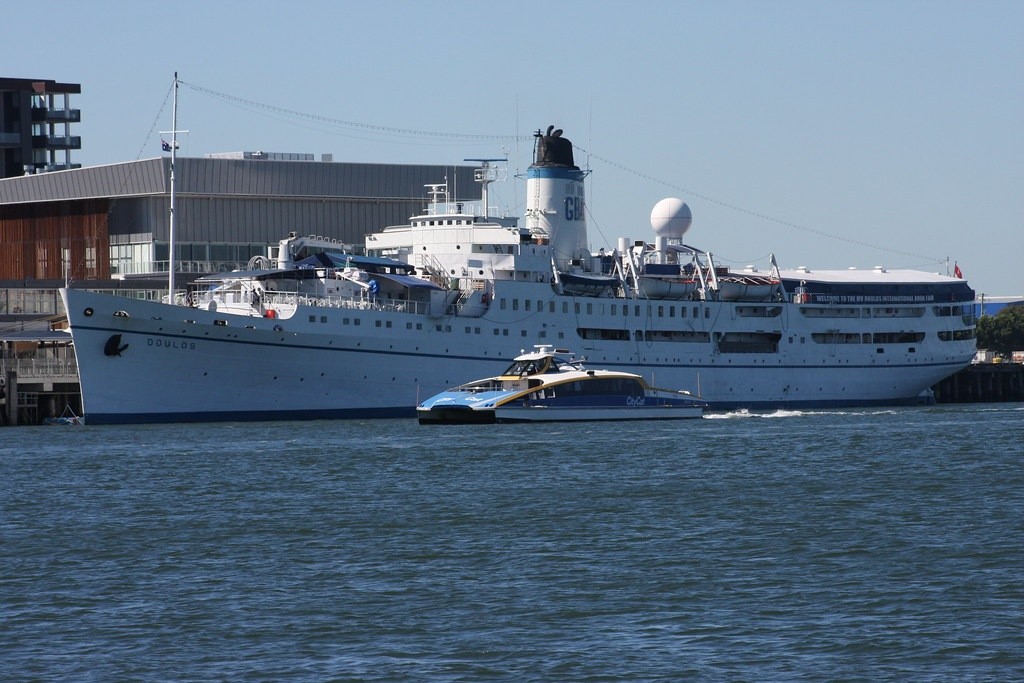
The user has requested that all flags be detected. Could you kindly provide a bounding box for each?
[955,264,962,279]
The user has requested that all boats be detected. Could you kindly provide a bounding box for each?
[415,343,709,426]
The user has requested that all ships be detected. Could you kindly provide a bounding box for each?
[58,68,980,427]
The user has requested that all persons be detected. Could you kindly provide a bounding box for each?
[242,287,276,307]
